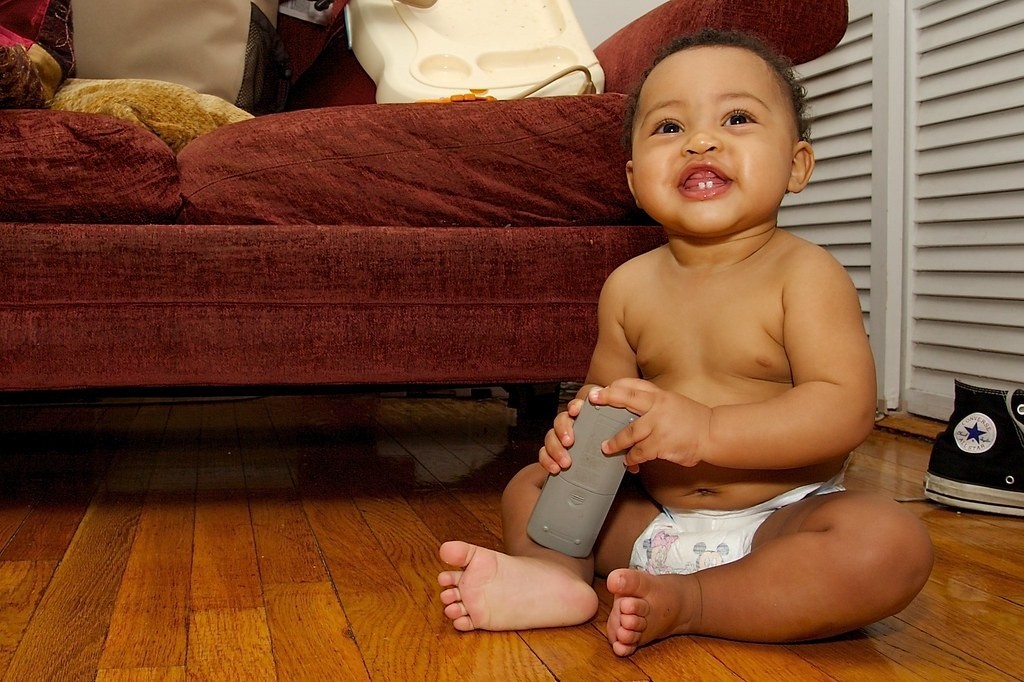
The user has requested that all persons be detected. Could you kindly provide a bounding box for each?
[437,29,936,657]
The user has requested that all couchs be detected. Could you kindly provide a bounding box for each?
[0,1,849,441]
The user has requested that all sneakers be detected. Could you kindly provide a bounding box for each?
[923,378,1024,517]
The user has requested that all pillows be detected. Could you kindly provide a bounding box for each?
[49,76,256,155]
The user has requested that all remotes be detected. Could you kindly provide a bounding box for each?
[526,387,641,557]
